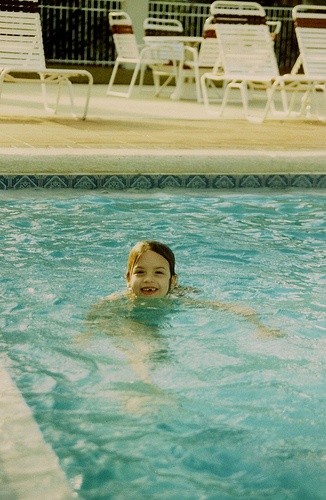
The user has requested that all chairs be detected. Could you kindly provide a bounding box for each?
[201,1,326,125]
[144,18,192,96]
[107,12,169,98]
[207,21,281,98]
[170,17,246,100]
[0,0,94,121]
[267,5,326,121]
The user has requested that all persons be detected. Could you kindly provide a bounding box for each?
[106,239,179,299]
